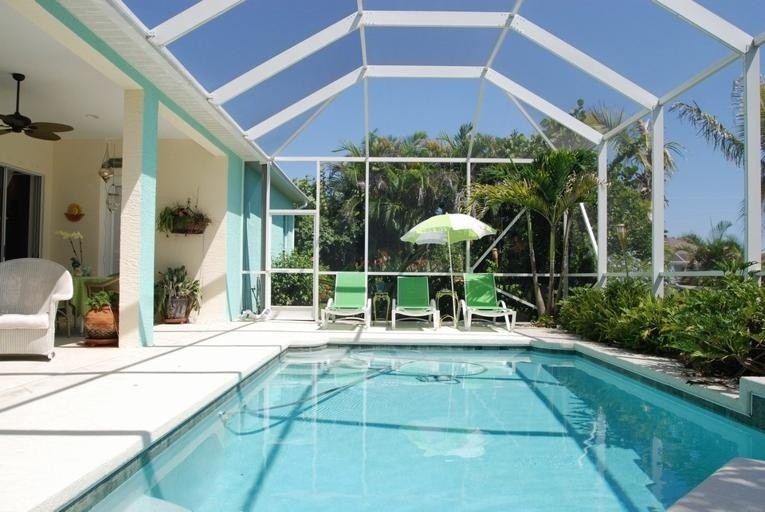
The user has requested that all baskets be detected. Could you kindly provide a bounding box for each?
[170,208,208,234]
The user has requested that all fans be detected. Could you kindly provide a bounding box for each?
[0,72,74,141]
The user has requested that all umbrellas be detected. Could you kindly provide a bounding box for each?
[401,212,497,328]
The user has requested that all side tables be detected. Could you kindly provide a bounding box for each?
[373,292,391,321]
[435,290,459,328]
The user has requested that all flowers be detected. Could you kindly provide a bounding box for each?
[55,228,88,265]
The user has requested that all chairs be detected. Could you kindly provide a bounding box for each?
[0,257,75,360]
[320,271,372,331]
[85,272,119,296]
[391,276,440,330]
[56,301,71,337]
[460,272,517,331]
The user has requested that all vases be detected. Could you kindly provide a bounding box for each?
[70,258,81,270]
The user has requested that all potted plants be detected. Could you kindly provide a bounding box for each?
[154,265,205,324]
[83,291,118,348]
[157,200,211,238]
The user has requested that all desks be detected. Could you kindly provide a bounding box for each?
[72,276,113,337]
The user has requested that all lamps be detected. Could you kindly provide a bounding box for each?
[98,142,114,183]
